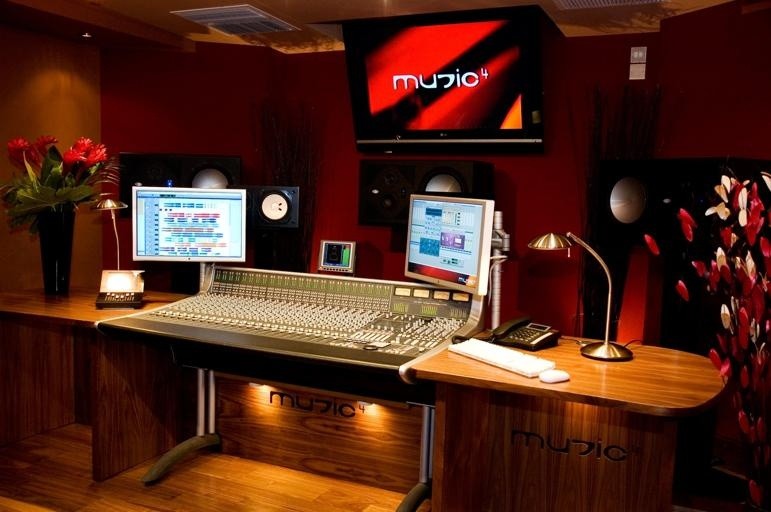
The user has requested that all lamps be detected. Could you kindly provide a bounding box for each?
[528,232,633,361]
[89,198,128,270]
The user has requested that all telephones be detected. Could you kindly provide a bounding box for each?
[493,318,560,351]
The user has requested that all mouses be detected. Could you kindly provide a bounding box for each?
[538,369,570,382]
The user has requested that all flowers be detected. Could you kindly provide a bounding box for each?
[0,134,119,242]
[644,174,771,511]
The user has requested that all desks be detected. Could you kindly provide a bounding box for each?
[0,288,192,482]
[407,329,729,512]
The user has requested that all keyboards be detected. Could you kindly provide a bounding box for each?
[448,337,556,380]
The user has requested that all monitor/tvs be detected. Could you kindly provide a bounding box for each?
[131,186,246,262]
[404,194,495,296]
[341,6,544,154]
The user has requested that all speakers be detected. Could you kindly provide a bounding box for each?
[358,160,493,228]
[119,152,241,218]
[600,160,656,257]
[242,183,300,230]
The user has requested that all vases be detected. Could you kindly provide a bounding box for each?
[38,212,74,294]
